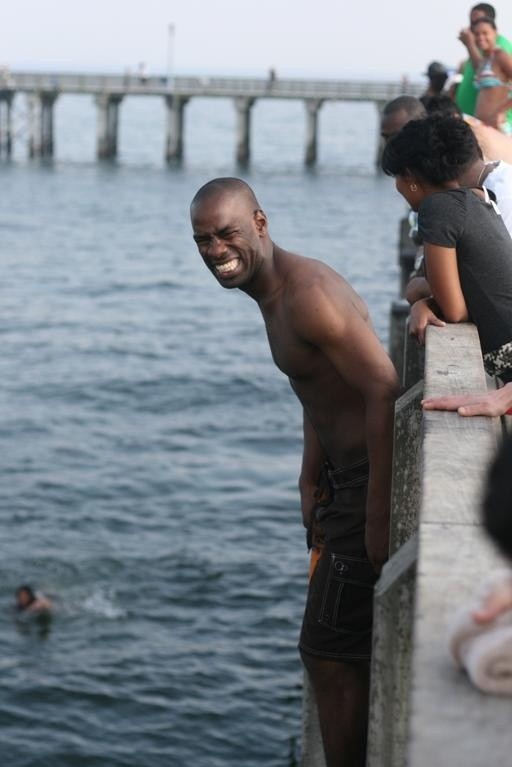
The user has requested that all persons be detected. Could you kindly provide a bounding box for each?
[6,581,59,620]
[187,171,403,767]
[464,416,512,632]
